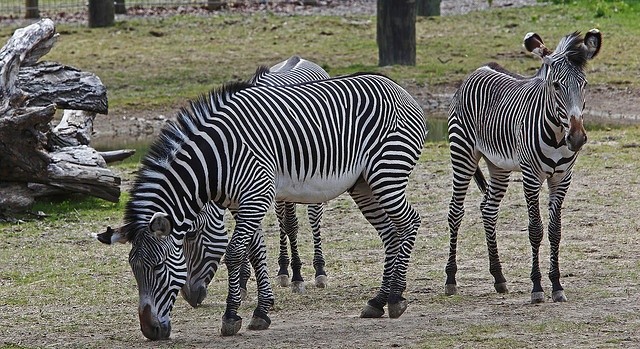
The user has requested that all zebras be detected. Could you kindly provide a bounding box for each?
[179,55,332,309]
[95,71,428,342]
[444,26,602,304]
